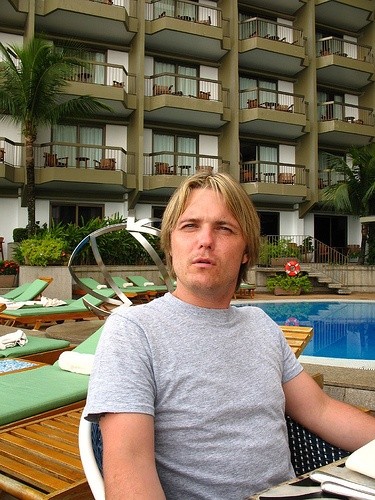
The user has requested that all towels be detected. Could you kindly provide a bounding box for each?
[41,297,66,308]
[58,350,95,374]
[1,329,26,351]
[0,296,41,311]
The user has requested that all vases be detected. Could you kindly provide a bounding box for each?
[0,275,17,287]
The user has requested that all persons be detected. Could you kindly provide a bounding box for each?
[82,171,375,500]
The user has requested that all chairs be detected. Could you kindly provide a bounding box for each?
[43,152,69,168]
[247,98,293,113]
[0,275,314,500]
[94,158,117,169]
[79,405,348,499]
[154,162,176,175]
[278,173,297,184]
[190,91,211,101]
[152,84,173,96]
[249,31,298,44]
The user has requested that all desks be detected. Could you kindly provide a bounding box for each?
[264,173,275,183]
[268,36,279,41]
[179,165,191,176]
[75,157,90,169]
[181,16,191,22]
[343,117,354,122]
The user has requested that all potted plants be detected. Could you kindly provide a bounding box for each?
[265,274,312,296]
[299,236,315,263]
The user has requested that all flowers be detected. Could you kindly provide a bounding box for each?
[0,259,19,275]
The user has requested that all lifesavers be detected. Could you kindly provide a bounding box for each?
[285,261,300,276]
